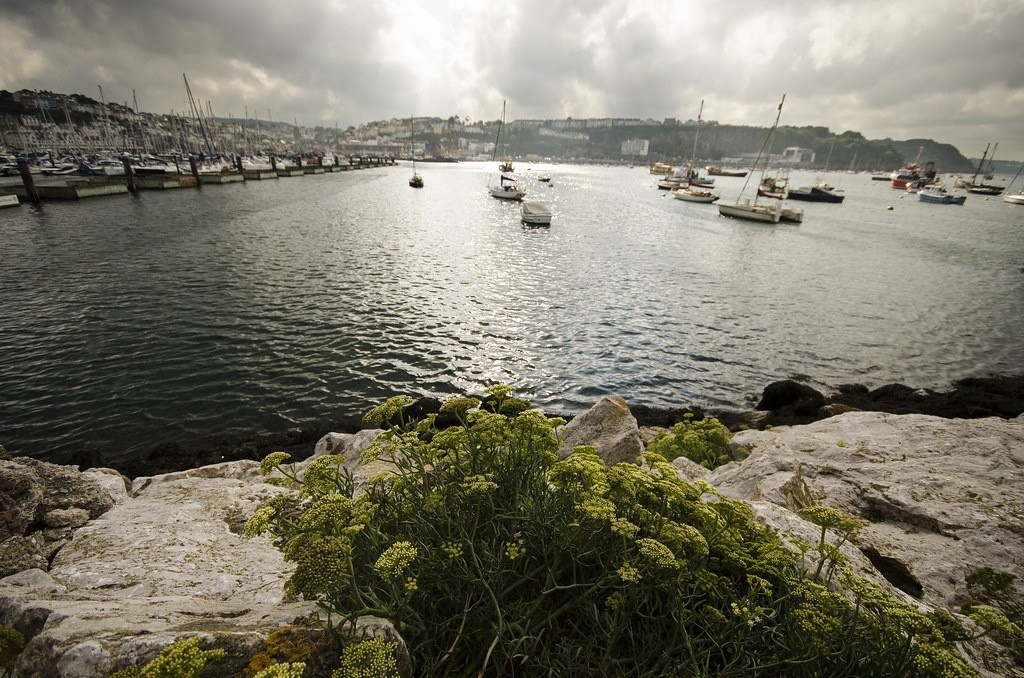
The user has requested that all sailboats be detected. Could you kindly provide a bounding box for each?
[953,142,991,189]
[657,97,716,189]
[487,100,526,200]
[718,93,782,224]
[0,67,399,207]
[1002,164,1024,204]
[821,140,885,175]
[963,142,1007,196]
[408,113,424,188]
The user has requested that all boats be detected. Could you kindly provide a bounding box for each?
[549,183,553,187]
[871,176,890,181]
[537,175,551,182]
[759,165,790,199]
[891,146,939,190]
[702,163,748,176]
[906,168,947,194]
[787,176,845,204]
[649,161,672,175]
[918,176,968,205]
[670,187,721,203]
[498,159,515,173]
[519,201,552,224]
[759,202,806,224]
[528,168,531,170]
